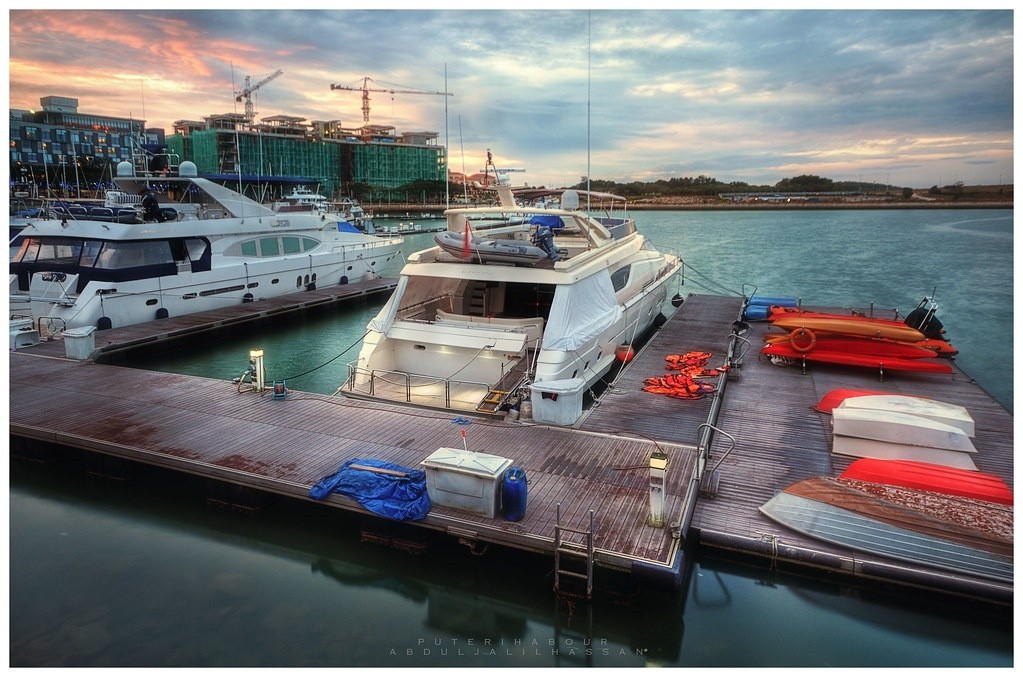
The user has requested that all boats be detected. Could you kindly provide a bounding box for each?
[336,10,688,429]
[742,293,1014,583]
[9,136,403,350]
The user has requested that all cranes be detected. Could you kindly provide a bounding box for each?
[235,68,285,131]
[330,77,454,125]
